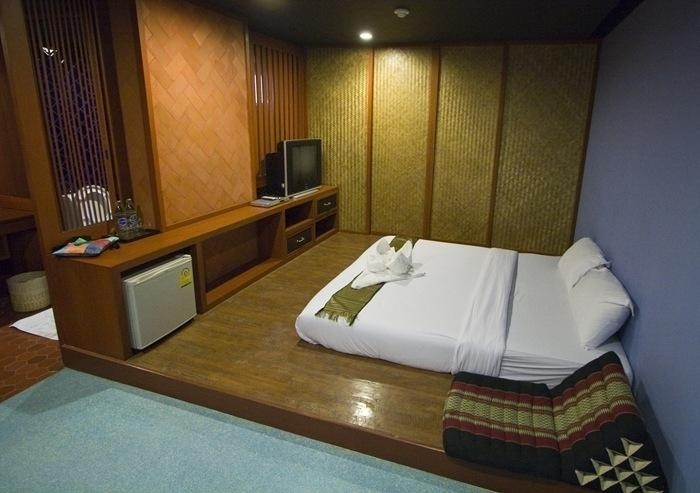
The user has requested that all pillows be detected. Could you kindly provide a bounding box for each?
[559,236,629,285]
[566,272,635,354]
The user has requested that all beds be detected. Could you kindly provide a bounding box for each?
[295,235,635,390]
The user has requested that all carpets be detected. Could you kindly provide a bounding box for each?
[2,363,492,492]
[12,308,70,341]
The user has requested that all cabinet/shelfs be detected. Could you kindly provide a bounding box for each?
[49,184,340,363]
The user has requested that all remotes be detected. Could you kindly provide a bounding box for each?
[261,195,277,200]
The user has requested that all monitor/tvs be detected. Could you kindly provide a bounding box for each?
[266,139,321,197]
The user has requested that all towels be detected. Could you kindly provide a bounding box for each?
[351,237,426,289]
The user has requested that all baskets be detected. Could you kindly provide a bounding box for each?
[6,271,51,312]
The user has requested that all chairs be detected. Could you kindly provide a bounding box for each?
[445,352,669,493]
